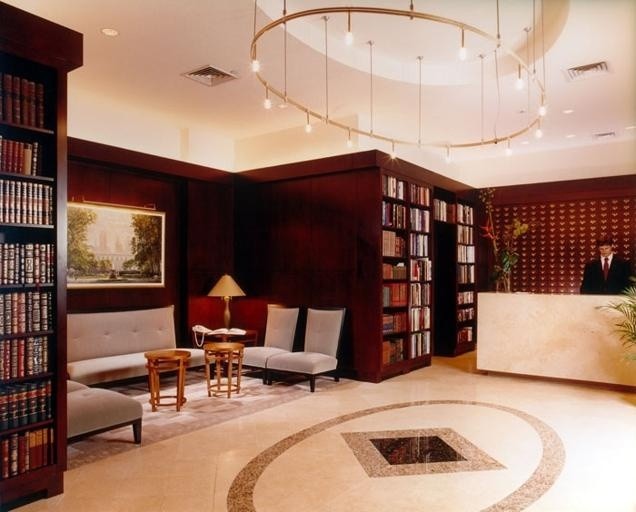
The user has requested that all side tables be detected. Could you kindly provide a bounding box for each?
[191,329,258,377]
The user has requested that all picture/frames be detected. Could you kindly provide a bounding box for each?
[67,201,165,288]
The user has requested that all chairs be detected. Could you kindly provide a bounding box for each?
[67,388,143,444]
[266,304,347,392]
[238,305,300,386]
[67,378,88,393]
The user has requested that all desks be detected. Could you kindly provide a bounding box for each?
[144,349,191,412]
[203,342,245,397]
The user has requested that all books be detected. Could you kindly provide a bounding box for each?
[457,202,473,343]
[381,174,431,366]
[0,69,57,481]
[431,196,457,223]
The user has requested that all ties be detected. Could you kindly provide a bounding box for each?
[603,258,609,282]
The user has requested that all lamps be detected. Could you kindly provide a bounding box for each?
[206,274,246,329]
[250,0,548,160]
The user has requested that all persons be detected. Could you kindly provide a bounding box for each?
[580,237,631,295]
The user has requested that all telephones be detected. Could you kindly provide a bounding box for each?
[192,325,212,333]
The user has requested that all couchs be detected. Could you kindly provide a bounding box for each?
[67,304,205,386]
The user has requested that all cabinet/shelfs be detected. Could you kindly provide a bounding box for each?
[458,223,475,326]
[410,203,431,333]
[383,196,406,340]
[0,120,56,438]
